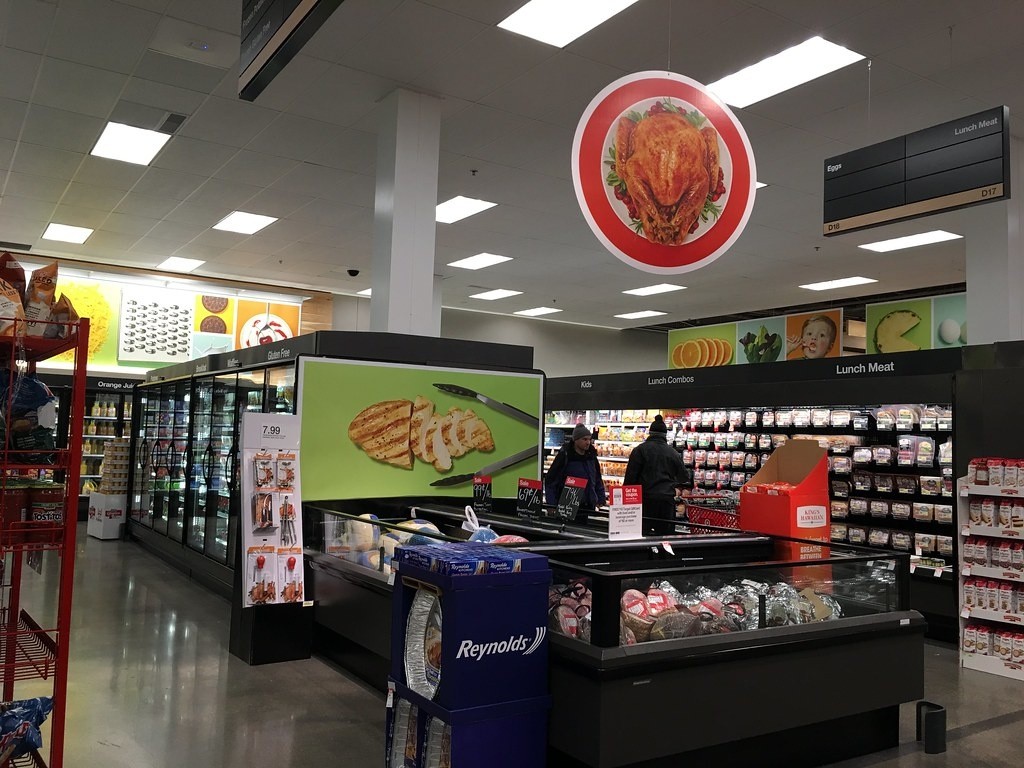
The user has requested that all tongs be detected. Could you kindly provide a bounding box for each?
[430,383,539,486]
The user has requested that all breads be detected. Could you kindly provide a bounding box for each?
[545,583,836,645]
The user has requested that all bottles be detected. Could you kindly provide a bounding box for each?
[975,461,989,484]
[68,401,133,494]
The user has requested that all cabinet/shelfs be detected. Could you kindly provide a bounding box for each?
[0,317,89,768]
[957,476,1024,682]
[542,423,681,511]
[52,388,285,521]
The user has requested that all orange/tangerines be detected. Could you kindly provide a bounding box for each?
[672,338,733,368]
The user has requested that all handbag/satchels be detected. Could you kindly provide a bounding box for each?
[544,450,568,504]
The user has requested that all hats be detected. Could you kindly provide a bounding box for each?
[572,423,591,441]
[649,415,667,437]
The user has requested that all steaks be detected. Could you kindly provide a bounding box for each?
[347,395,495,469]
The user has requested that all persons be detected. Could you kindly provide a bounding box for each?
[623,414,689,536]
[786,314,837,359]
[545,423,606,520]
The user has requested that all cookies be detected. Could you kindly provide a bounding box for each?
[200,296,228,335]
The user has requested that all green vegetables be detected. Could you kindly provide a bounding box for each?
[603,97,723,235]
[738,325,783,364]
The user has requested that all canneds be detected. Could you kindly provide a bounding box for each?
[100,437,129,494]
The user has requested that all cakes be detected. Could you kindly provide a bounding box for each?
[875,309,921,353]
[240,313,293,348]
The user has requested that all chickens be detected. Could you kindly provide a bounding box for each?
[615,112,719,245]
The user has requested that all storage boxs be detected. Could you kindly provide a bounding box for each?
[968,457,1024,487]
[963,536,1024,572]
[968,498,1024,530]
[963,578,1024,614]
[963,624,1024,663]
